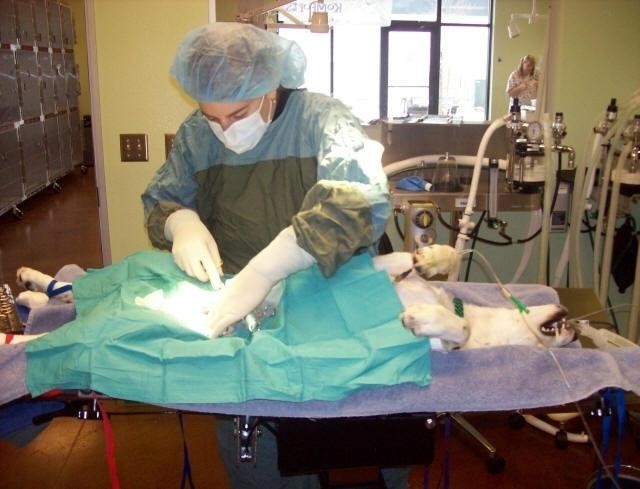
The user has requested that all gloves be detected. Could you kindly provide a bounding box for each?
[201,224,318,339]
[163,208,225,290]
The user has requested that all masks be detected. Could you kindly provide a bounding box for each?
[201,95,276,154]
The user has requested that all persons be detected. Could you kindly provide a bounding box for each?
[505,54,542,117]
[137,23,414,488]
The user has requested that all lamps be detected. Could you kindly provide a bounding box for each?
[508,1,549,40]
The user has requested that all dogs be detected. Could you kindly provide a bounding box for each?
[15,244,580,354]
